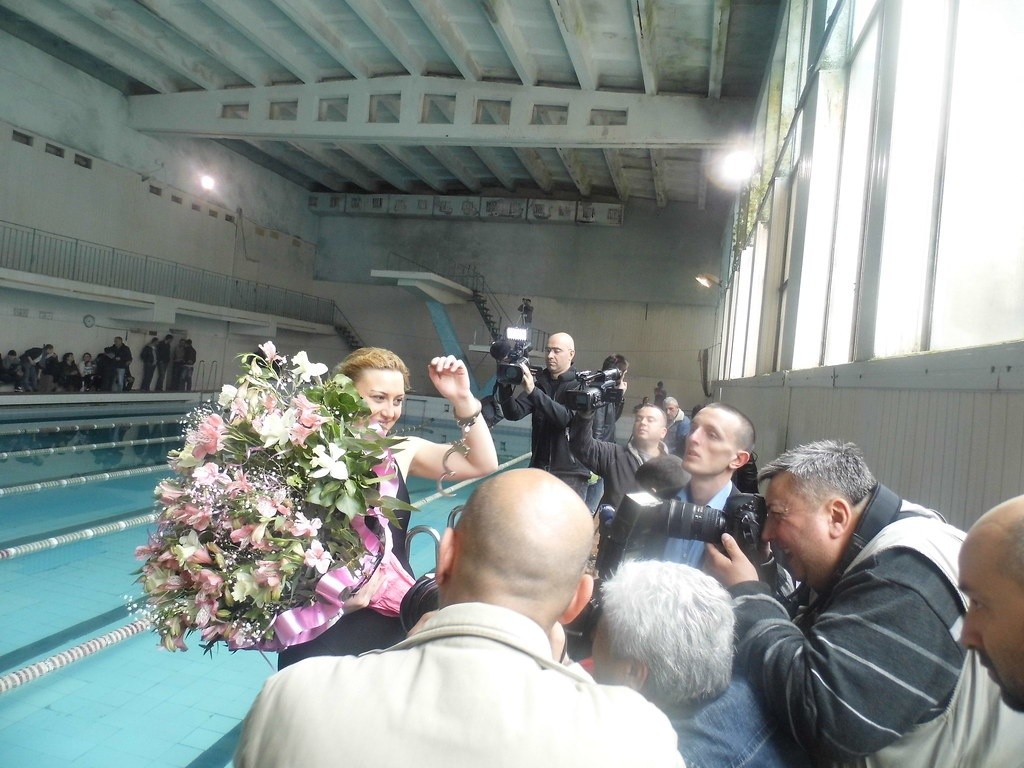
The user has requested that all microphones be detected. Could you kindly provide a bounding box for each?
[490,341,511,360]
[558,372,575,382]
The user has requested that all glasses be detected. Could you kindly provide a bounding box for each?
[543,347,572,354]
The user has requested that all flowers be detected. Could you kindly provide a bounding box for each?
[130,337,421,671]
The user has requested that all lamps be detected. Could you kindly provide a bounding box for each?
[695,272,727,289]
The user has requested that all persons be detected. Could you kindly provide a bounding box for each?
[497,332,592,502]
[634,397,653,410]
[179,339,196,391]
[592,559,787,768]
[692,405,707,419]
[569,404,666,570]
[155,335,173,391]
[644,403,757,570]
[0,336,135,393]
[278,347,498,671]
[959,495,1024,713]
[235,468,686,768]
[141,337,158,392]
[653,381,666,407]
[661,397,690,459]
[518,299,534,328]
[586,354,628,514]
[705,439,1024,768]
[170,339,186,390]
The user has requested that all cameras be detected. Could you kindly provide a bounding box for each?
[655,492,768,561]
[398,573,443,632]
[562,488,659,663]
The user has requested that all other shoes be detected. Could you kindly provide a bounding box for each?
[14,386,23,392]
